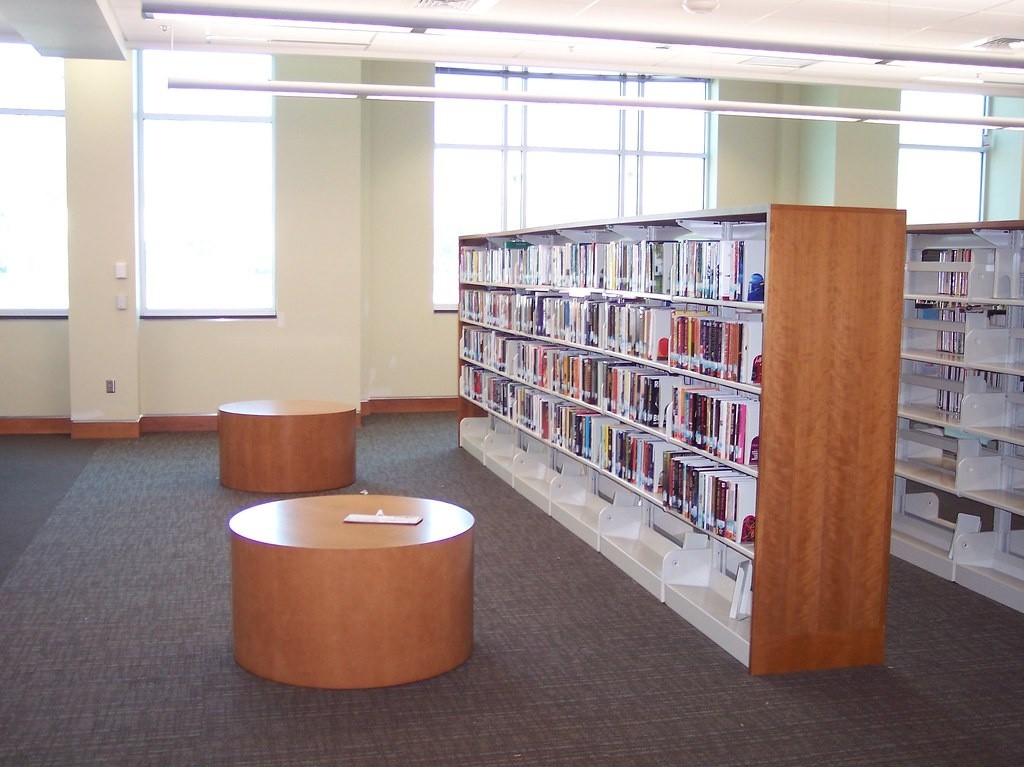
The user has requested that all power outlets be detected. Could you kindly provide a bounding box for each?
[106,379,116,393]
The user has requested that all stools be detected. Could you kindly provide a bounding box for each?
[228,494,477,690]
[217,399,357,494]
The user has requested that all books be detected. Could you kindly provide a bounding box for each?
[459,241,765,542]
[937,249,1006,413]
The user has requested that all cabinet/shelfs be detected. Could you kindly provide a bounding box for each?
[458,203,907,677]
[890,220,1024,613]
